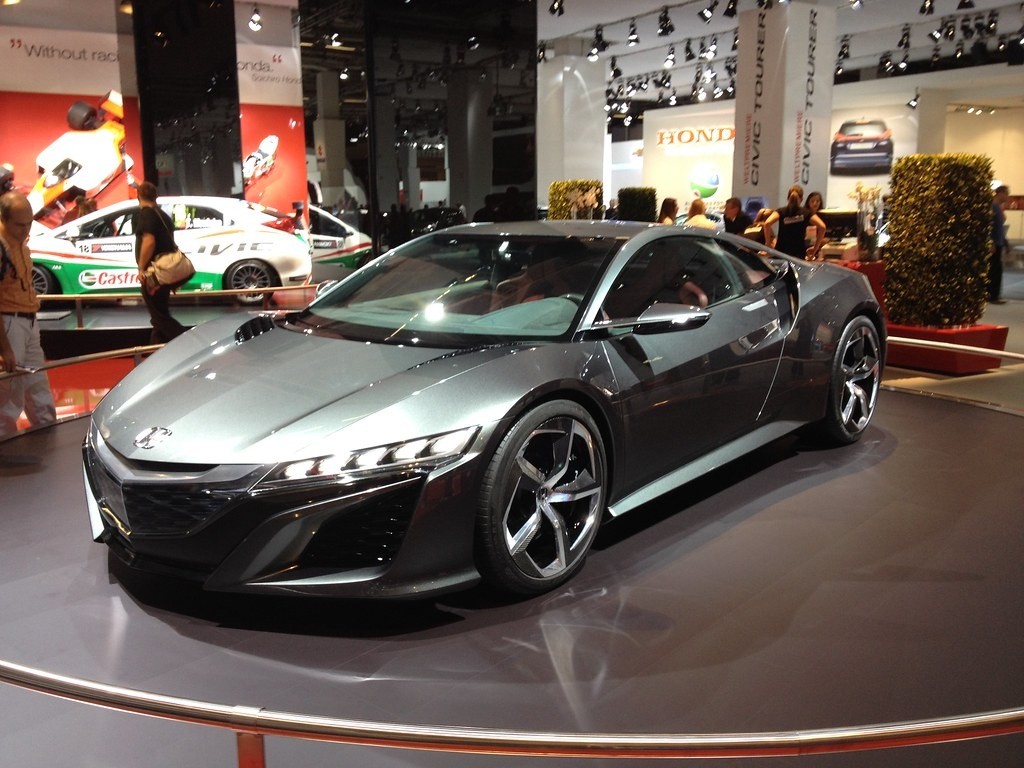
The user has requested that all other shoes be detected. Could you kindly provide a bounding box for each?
[987,296,1006,304]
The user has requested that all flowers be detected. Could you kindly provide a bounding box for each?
[849,183,889,261]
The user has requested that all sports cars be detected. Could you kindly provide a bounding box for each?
[81,220,889,611]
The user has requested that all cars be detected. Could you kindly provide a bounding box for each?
[26,194,315,309]
[405,207,466,242]
[306,199,373,268]
[830,118,895,174]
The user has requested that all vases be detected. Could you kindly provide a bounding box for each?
[834,258,891,317]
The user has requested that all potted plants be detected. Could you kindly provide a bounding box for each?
[874,155,1009,373]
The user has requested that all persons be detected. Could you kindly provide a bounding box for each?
[386,185,525,249]
[130,180,185,346]
[653,185,1015,303]
[0,187,99,439]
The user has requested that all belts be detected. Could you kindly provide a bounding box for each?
[0,310,37,319]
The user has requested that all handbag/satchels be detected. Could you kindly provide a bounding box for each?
[152,250,196,290]
